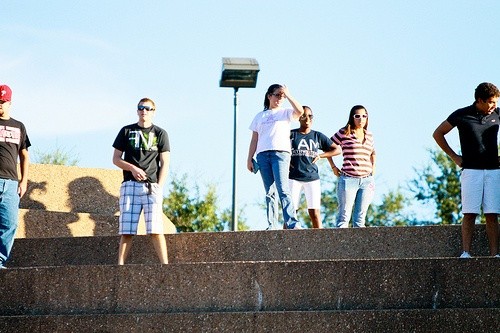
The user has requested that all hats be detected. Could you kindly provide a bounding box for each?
[0,85,12,102]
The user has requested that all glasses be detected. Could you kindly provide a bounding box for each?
[353,114,367,119]
[300,114,313,120]
[138,105,155,111]
[272,93,286,99]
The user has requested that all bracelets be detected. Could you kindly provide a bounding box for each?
[331,166,336,168]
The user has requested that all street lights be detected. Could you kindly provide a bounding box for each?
[221,57,260,231]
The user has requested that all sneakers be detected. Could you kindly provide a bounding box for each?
[495,254,500,257]
[460,252,471,258]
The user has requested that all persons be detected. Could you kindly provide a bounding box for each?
[247,84,305,230]
[433,82,500,258]
[112,98,170,266]
[284,106,343,229]
[328,106,376,229]
[0,84,31,270]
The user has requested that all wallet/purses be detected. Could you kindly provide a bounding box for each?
[251,158,260,174]
